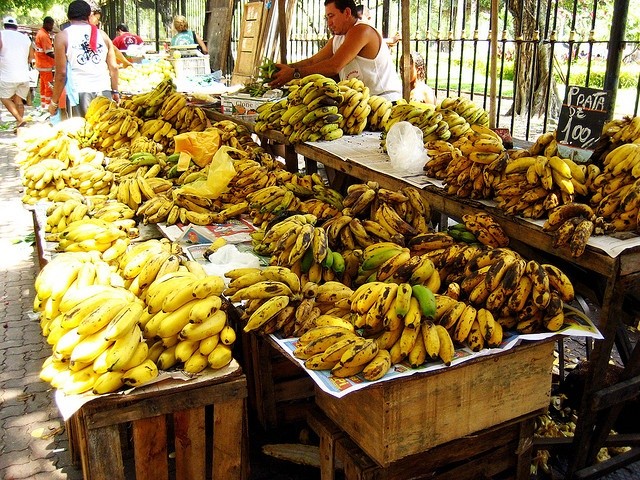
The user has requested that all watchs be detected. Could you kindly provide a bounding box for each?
[294,69,302,79]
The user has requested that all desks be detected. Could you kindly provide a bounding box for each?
[207,106,295,172]
[66,360,248,480]
[295,146,640,479]
[229,308,314,429]
[308,410,543,479]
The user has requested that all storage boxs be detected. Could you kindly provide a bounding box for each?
[315,339,562,466]
[169,57,210,75]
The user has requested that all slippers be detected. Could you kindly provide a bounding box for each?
[14,121,26,132]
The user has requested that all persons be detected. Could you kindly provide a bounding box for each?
[112,23,144,49]
[172,15,207,53]
[399,51,434,105]
[267,0,403,103]
[88,2,101,25]
[49,0,119,116]
[0,16,34,130]
[34,17,55,112]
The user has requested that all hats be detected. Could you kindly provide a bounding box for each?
[3,16,18,25]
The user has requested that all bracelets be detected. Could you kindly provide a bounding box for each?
[112,90,119,94]
[50,100,59,108]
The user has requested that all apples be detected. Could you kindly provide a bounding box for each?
[235,105,246,114]
[247,109,256,115]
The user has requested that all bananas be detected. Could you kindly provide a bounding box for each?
[366,96,509,200]
[223,181,574,383]
[32,238,236,397]
[255,73,372,145]
[15,122,114,207]
[80,75,207,174]
[115,119,323,226]
[494,114,640,259]
[45,188,139,255]
